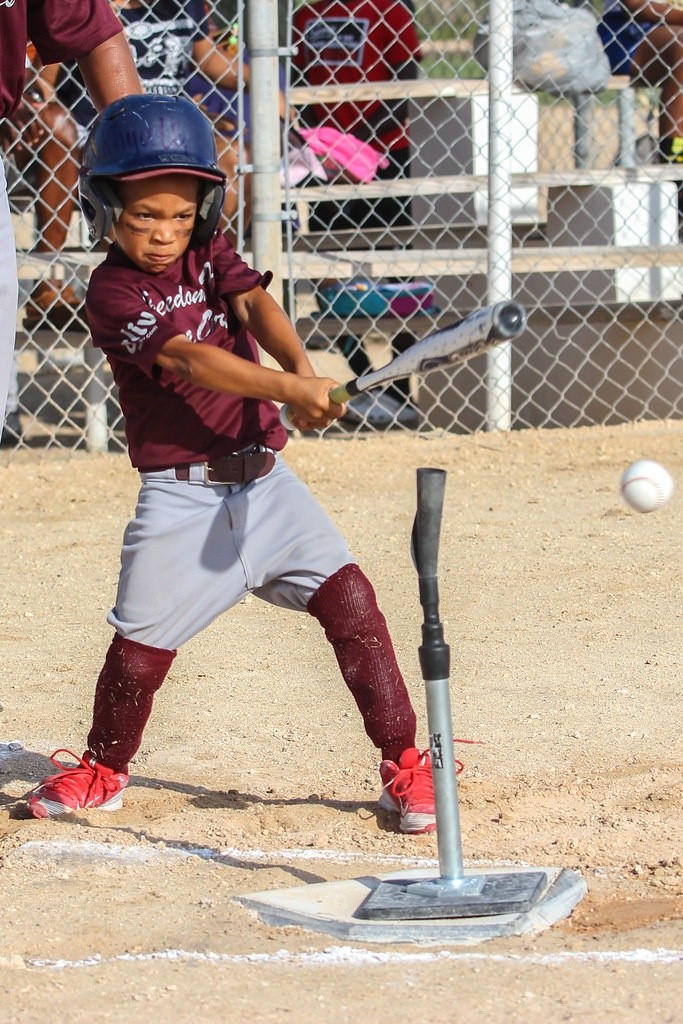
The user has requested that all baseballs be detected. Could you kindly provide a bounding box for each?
[619,459,675,513]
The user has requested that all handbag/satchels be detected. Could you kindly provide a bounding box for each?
[473,0,611,94]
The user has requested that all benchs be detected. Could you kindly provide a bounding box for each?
[11,73,683,455]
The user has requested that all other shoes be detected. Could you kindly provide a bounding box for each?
[23,284,90,333]
[342,389,419,427]
[1,412,22,447]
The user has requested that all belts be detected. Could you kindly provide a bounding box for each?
[175,444,277,486]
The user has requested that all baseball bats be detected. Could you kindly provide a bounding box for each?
[280,301,530,433]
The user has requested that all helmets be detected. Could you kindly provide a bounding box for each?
[77,93,228,246]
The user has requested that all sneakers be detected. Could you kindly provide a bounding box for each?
[378,738,481,835]
[25,749,129,818]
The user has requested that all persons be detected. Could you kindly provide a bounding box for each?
[0,0,422,424]
[596,0,683,164]
[0,0,143,446]
[15,94,449,833]
